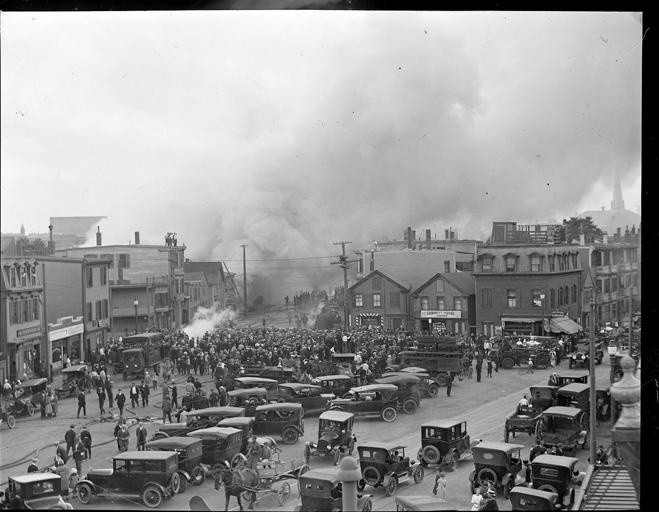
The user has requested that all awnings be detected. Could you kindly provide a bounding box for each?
[551,316,583,334]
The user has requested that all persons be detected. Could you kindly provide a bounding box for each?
[148,323,499,383]
[247,436,272,468]
[519,394,544,413]
[597,320,641,362]
[503,335,578,373]
[471,441,564,511]
[77,389,86,418]
[548,373,558,387]
[2,374,28,397]
[433,469,447,499]
[599,444,608,465]
[114,420,147,452]
[163,232,177,247]
[304,422,342,465]
[446,372,453,396]
[161,376,226,423]
[67,355,71,367]
[39,388,57,417]
[388,450,400,463]
[29,425,94,500]
[89,328,156,417]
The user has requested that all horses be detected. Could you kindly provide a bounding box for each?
[215,469,259,511]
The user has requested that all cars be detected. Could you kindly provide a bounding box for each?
[15,378,49,409]
[54,365,92,399]
[222,334,466,452]
[417,417,481,472]
[296,468,374,511]
[356,442,425,494]
[509,454,586,510]
[303,410,357,466]
[394,495,456,512]
[487,315,640,456]
[469,442,524,499]
[1,398,34,430]
[0,472,74,510]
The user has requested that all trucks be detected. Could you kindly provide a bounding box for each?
[123,332,162,378]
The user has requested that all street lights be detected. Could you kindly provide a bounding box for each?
[238,244,249,314]
[134,297,139,334]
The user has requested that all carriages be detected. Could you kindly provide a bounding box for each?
[214,460,310,512]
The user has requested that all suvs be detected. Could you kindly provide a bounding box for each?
[72,400,304,494]
[74,448,184,511]
